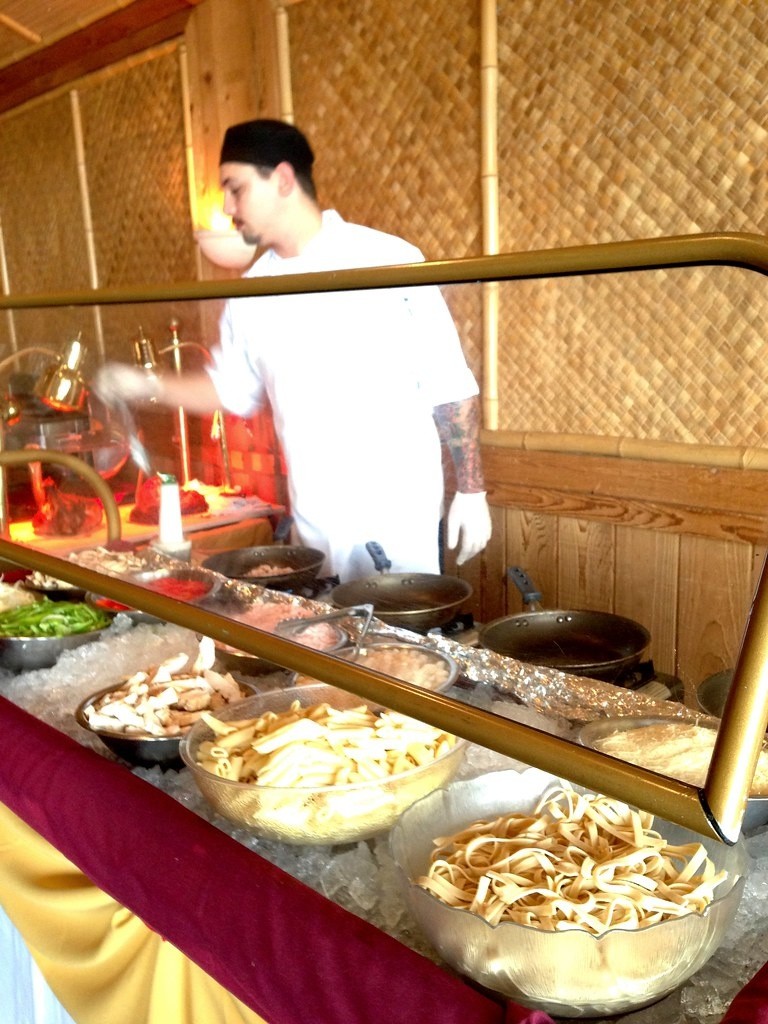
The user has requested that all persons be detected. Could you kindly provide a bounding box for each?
[91,117,492,587]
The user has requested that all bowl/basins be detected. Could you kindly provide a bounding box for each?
[576,715,768,834]
[0,547,220,669]
[290,642,459,695]
[388,766,750,1014]
[74,672,263,769]
[180,682,470,846]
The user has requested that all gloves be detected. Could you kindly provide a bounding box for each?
[447,489,491,566]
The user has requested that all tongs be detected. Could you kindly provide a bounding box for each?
[275,604,376,662]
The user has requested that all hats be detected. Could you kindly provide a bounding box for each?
[219,117,316,172]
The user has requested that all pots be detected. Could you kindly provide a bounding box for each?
[328,540,471,630]
[203,513,325,590]
[478,566,651,677]
[20,406,95,493]
